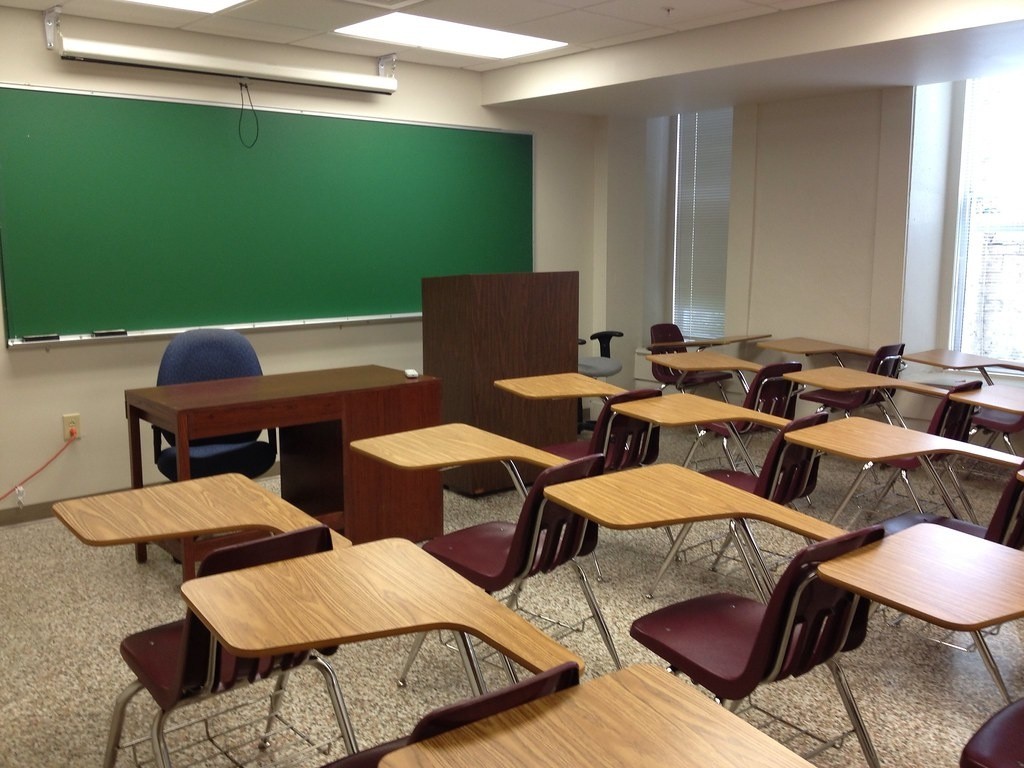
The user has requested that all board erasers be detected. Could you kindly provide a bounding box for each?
[23,334,59,342]
[92,329,127,337]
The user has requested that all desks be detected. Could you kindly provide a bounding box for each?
[124,364,446,582]
[815,522,1023,703]
[51,472,356,568]
[644,331,1023,428]
[492,373,630,449]
[376,662,820,768]
[783,415,1024,522]
[179,535,584,755]
[348,421,577,504]
[543,462,855,599]
[611,393,795,477]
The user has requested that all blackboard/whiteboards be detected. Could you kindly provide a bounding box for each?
[0,83,535,349]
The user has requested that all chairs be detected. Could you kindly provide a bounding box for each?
[965,406,1023,480]
[870,460,1024,654]
[649,323,734,446]
[536,389,683,584]
[799,342,906,422]
[676,411,829,588]
[577,331,624,380]
[317,659,581,767]
[958,697,1024,767]
[151,328,279,483]
[628,524,888,768]
[394,452,623,699]
[101,524,362,767]
[827,379,983,526]
[682,361,818,511]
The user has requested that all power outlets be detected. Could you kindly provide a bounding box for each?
[61,413,82,442]
